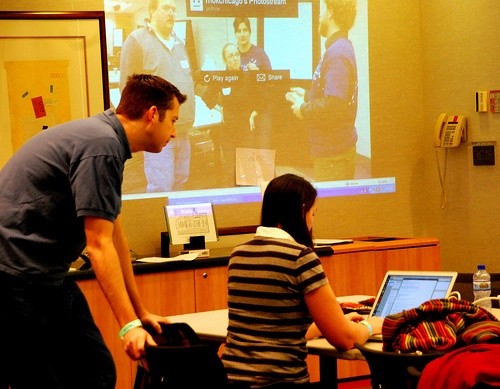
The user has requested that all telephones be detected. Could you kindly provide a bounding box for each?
[433,113,464,149]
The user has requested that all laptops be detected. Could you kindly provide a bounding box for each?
[365,271,458,340]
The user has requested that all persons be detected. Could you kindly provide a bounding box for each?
[216,173,384,389]
[232,17,272,71]
[118,0,195,196]
[289,0,358,180]
[0,73,189,389]
[222,43,241,71]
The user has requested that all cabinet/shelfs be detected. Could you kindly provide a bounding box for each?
[69,234,442,389]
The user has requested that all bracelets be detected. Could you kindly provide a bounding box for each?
[118,319,144,340]
[359,320,373,337]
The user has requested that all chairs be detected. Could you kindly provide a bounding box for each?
[353,340,445,389]
[133,322,229,389]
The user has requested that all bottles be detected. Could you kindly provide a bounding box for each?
[472,265,492,311]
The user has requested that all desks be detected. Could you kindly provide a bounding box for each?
[164,292,500,389]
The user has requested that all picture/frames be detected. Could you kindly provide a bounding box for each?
[0,10,111,172]
